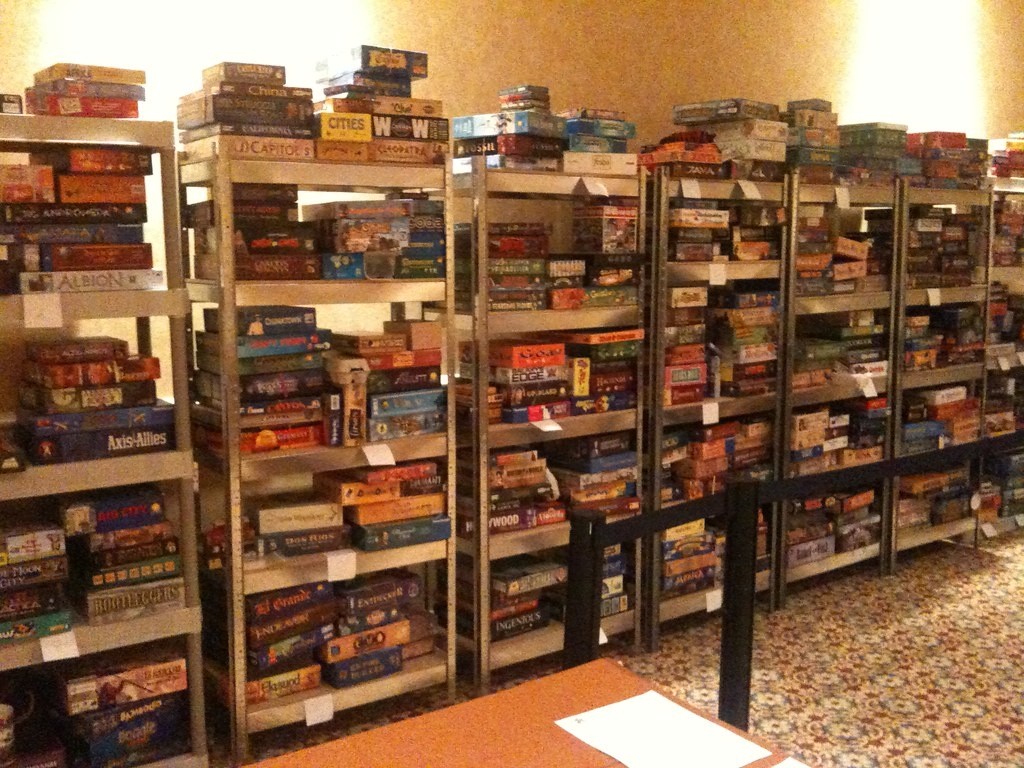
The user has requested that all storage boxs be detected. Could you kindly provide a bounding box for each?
[0,44,1023,768]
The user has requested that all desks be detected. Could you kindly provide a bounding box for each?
[236,657,814,766]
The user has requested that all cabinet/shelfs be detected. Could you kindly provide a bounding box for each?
[651,162,791,654]
[978,177,1023,538]
[780,170,902,611]
[891,176,994,574]
[173,144,457,767]
[451,152,662,698]
[1,114,211,768]
